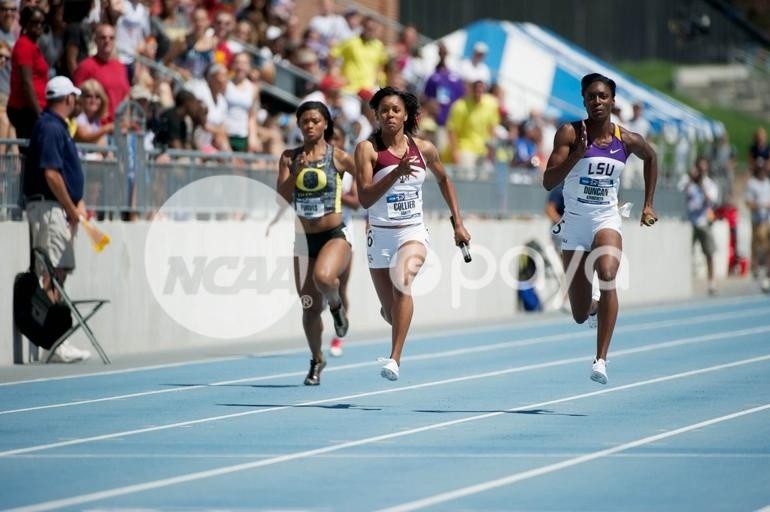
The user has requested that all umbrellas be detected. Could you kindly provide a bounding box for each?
[405,18,726,142]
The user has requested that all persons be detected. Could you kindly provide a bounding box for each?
[275,100,355,387]
[14,72,92,364]
[619,100,654,190]
[0,0,559,221]
[261,123,361,358]
[353,86,473,382]
[542,72,659,386]
[681,126,770,298]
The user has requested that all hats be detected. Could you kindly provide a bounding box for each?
[46,77,82,101]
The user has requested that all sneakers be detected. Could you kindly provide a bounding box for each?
[48,341,90,364]
[591,358,607,384]
[330,338,342,357]
[304,358,327,386]
[328,301,348,336]
[381,359,400,381]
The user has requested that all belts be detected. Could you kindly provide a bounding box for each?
[25,195,57,202]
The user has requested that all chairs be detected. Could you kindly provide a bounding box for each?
[32,245,113,366]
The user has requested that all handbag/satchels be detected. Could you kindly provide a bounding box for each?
[14,246,73,350]
